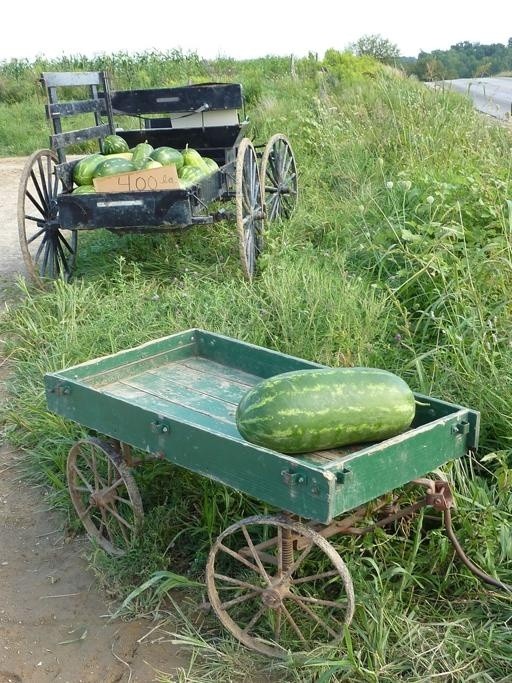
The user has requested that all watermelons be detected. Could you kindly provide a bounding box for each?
[71,135,219,195]
[236,367,417,455]
